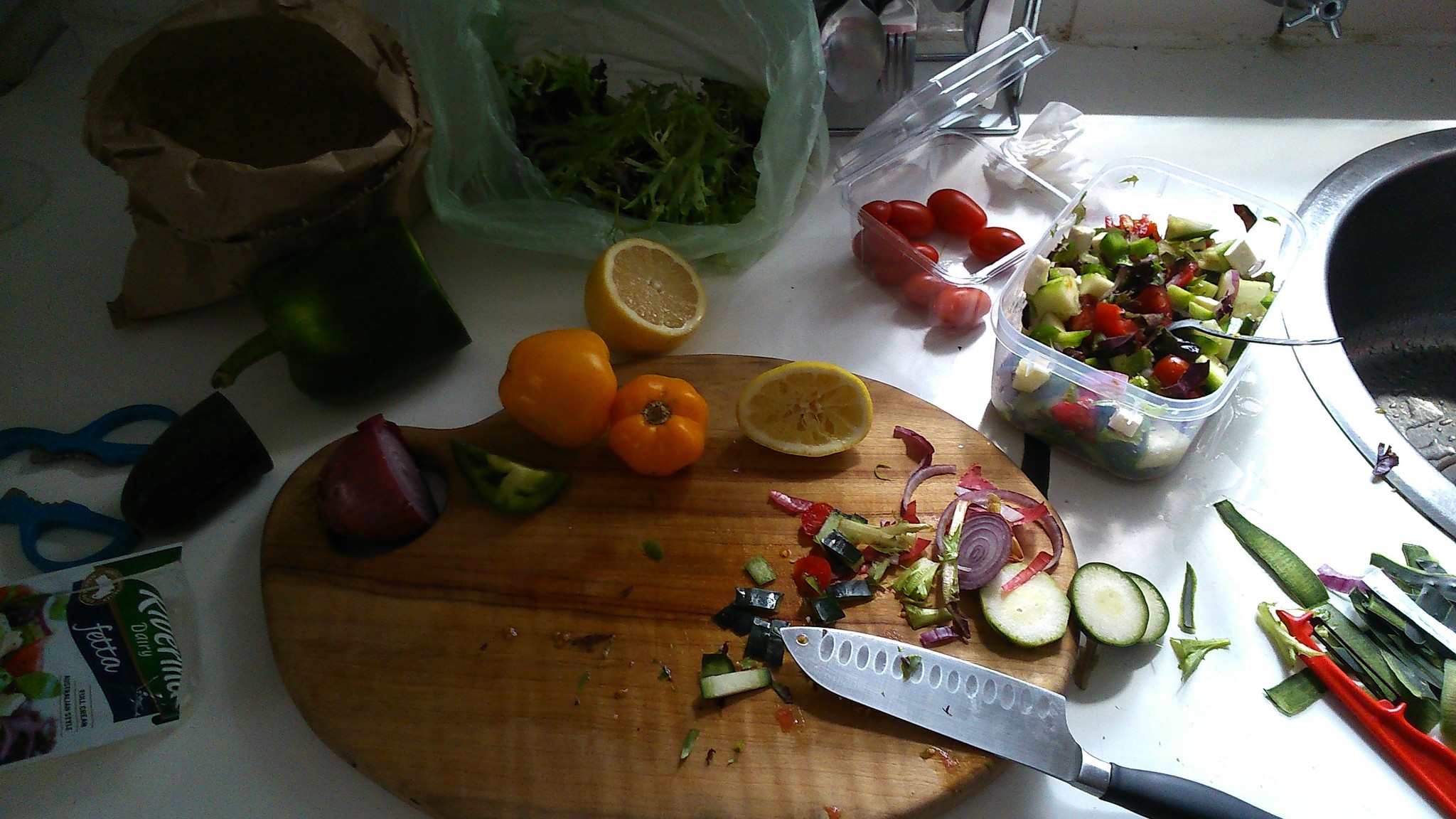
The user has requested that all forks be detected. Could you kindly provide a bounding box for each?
[879,0,918,102]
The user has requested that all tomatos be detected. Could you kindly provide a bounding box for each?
[1053,213,1213,443]
[853,185,1024,327]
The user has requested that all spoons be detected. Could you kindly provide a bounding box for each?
[820,0,887,104]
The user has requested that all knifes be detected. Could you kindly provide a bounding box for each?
[781,627,1282,819]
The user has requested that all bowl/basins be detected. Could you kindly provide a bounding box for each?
[840,131,1067,335]
[992,151,1308,484]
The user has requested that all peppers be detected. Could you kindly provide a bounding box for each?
[499,325,617,445]
[209,215,473,406]
[446,439,570,514]
[606,373,711,477]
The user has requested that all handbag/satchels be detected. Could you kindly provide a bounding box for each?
[390,1,829,280]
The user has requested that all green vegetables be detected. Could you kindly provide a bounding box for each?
[834,518,962,625]
[462,52,767,227]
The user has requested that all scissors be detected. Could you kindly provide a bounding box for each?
[1,404,180,572]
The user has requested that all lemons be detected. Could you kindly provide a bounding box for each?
[735,361,873,457]
[585,238,706,356]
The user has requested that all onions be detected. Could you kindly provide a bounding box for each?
[897,459,1065,641]
[314,416,436,555]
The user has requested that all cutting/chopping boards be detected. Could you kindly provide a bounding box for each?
[259,351,1081,819]
